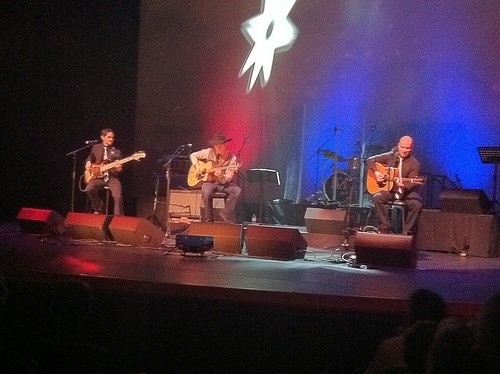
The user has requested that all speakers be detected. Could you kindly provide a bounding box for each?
[304,207,352,235]
[354,231,417,269]
[16,207,165,247]
[439,189,492,215]
[169,190,203,219]
[188,221,308,261]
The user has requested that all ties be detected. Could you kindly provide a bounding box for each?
[394,156,404,200]
[103,146,109,182]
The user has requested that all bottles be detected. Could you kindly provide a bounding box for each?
[252,214,256,224]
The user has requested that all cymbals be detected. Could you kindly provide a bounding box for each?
[322,150,344,162]
[365,145,385,149]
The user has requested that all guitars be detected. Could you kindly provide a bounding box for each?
[366,161,427,195]
[84,151,147,185]
[187,161,239,188]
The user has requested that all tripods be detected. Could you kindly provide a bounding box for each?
[477,146,500,215]
[146,176,163,230]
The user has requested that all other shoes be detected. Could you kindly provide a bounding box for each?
[93,204,102,214]
[205,218,214,223]
[220,212,233,223]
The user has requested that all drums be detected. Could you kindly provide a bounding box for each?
[323,172,353,204]
[348,158,361,170]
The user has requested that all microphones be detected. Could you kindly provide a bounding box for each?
[182,143,192,148]
[86,140,99,144]
[370,126,377,131]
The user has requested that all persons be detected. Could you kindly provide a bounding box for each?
[366,136,428,236]
[85,128,124,216]
[364,287,500,374]
[189,134,242,224]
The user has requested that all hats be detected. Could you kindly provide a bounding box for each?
[207,130,232,145]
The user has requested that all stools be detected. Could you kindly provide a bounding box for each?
[368,200,409,235]
[200,193,229,222]
[98,186,113,214]
